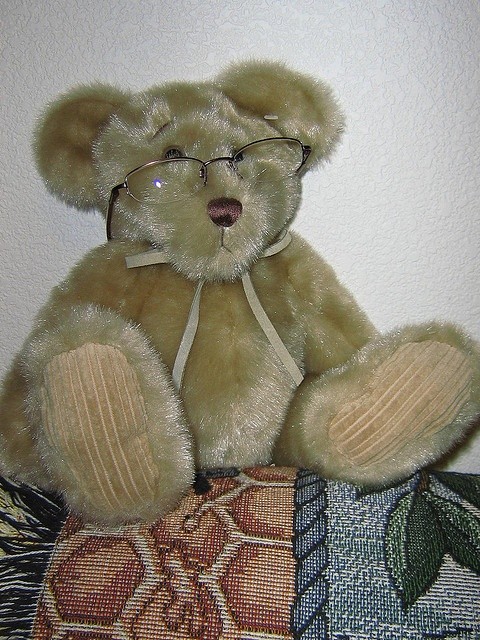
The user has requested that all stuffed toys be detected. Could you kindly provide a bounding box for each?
[0,59,480,528]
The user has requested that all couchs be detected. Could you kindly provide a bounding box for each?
[0,469,479,640]
